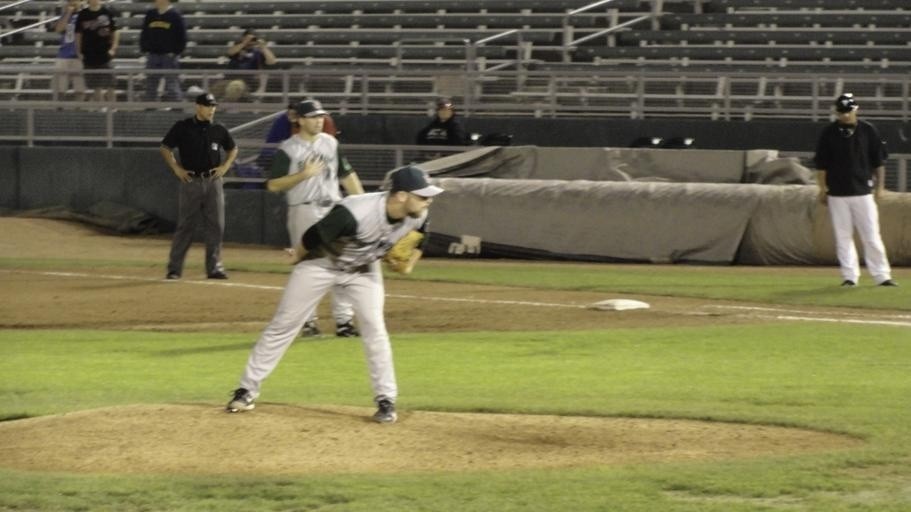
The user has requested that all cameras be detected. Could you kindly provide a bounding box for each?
[249,37,258,44]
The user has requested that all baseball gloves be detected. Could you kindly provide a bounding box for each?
[384,231,424,275]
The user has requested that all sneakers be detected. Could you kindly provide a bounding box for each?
[222,388,257,413]
[167,272,180,279]
[301,321,322,339]
[877,279,902,287]
[839,279,859,287]
[371,398,400,424]
[336,323,361,338]
[207,271,230,280]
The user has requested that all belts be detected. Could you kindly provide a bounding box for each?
[302,199,335,207]
[188,171,219,178]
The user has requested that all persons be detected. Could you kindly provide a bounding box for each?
[415,99,470,147]
[266,98,366,338]
[52,0,87,102]
[138,0,187,113]
[73,0,122,101]
[813,92,900,287]
[159,91,239,280]
[211,27,277,103]
[257,103,300,170]
[227,166,445,424]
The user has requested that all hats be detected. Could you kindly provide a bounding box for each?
[834,92,860,114]
[389,166,445,197]
[297,99,328,118]
[196,93,219,105]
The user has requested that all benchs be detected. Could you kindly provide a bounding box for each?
[0,0,910,121]
